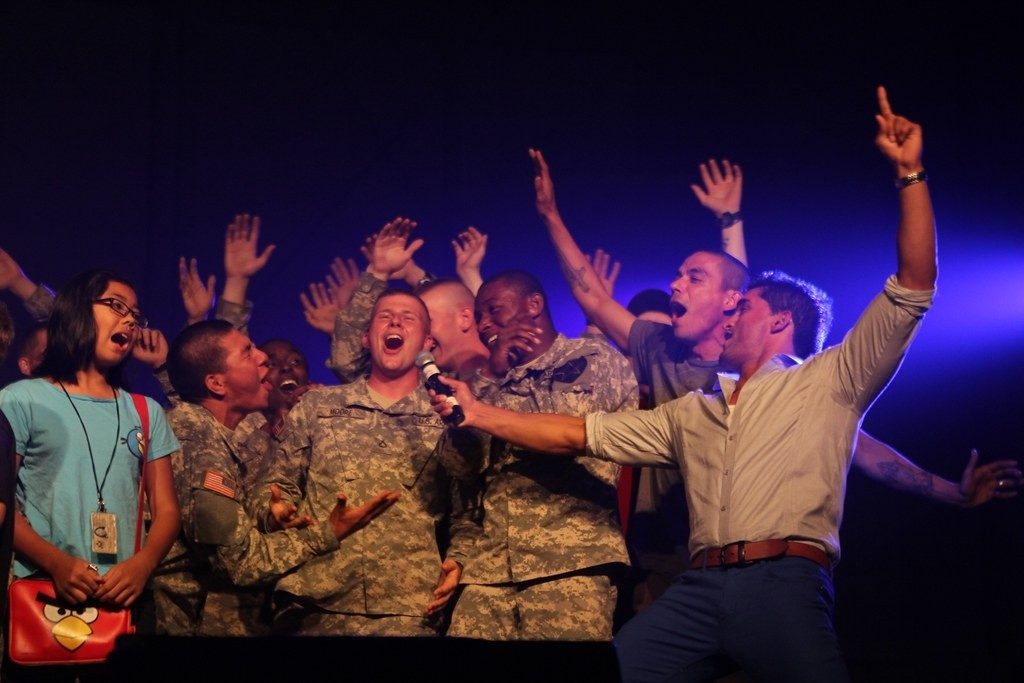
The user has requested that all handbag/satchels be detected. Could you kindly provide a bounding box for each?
[8,578,136,665]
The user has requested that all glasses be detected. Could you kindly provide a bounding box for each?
[94,297,149,330]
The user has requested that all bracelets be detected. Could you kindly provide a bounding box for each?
[894,167,927,188]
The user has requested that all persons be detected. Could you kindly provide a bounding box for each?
[428,86,937,683]
[1,301,16,627]
[0,267,180,628]
[214,213,344,415]
[452,159,751,541]
[144,318,402,636]
[435,270,639,640]
[330,217,620,381]
[528,149,1024,682]
[246,289,484,636]
[94,527,108,538]
[1,248,361,412]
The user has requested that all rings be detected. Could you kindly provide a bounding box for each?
[998,479,1005,489]
[88,564,98,572]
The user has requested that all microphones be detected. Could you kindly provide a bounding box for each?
[416,350,465,426]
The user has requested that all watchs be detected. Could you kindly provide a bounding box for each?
[717,211,742,228]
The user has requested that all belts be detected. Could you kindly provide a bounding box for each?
[693,537,830,571]
